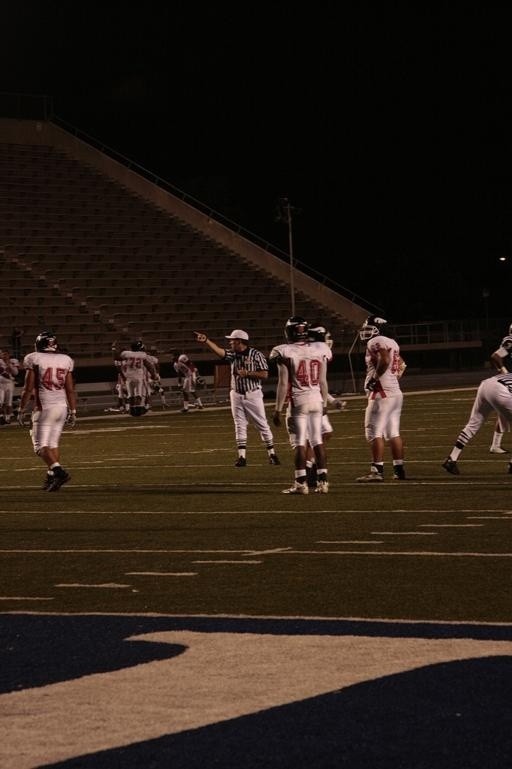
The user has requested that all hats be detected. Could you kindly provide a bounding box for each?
[225,330,249,340]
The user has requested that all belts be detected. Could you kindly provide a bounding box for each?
[231,387,259,395]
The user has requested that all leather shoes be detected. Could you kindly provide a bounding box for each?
[268,453,280,464]
[235,456,247,467]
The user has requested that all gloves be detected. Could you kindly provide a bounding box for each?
[366,376,377,391]
[16,408,26,427]
[272,410,282,428]
[331,399,353,410]
[323,407,328,416]
[65,409,77,428]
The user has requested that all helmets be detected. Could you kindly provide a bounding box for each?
[306,323,333,349]
[130,340,146,351]
[34,331,58,352]
[359,315,391,340]
[178,354,189,363]
[283,316,309,344]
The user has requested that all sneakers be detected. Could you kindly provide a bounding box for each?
[179,408,189,413]
[47,469,71,492]
[391,470,405,480]
[281,480,309,494]
[197,405,204,409]
[355,466,384,482]
[305,463,317,487]
[490,446,506,454]
[440,458,459,475]
[314,480,329,493]
[507,462,512,474]
[40,473,54,490]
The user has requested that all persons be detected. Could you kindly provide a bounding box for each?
[0,348,19,424]
[270,315,332,494]
[357,316,406,482]
[18,331,77,492]
[490,323,512,453]
[168,347,205,414]
[444,372,512,475]
[193,329,281,466]
[305,327,346,487]
[105,340,165,414]
